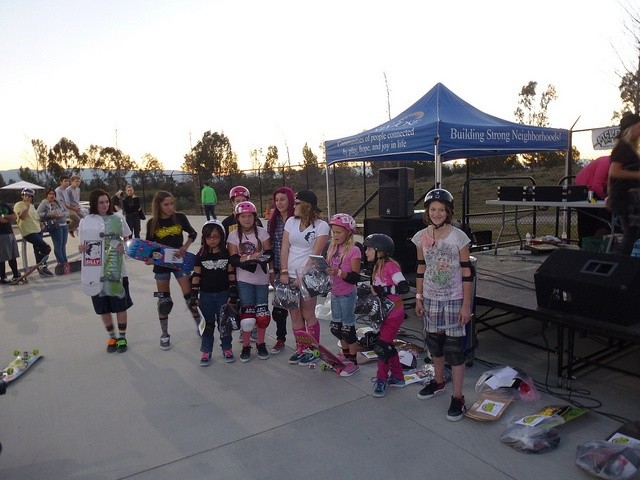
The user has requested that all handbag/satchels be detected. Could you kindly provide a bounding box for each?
[40,212,60,232]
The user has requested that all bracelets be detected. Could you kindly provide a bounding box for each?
[416,294,423,300]
[337,269,341,277]
[280,269,289,275]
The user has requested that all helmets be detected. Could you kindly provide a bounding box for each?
[230,186,251,198]
[202,219,226,241]
[328,213,356,233]
[363,233,395,256]
[234,201,258,219]
[424,189,454,208]
[21,188,35,197]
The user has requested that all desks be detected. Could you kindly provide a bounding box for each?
[485,200,627,257]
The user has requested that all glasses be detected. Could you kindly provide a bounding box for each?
[295,199,301,205]
[206,234,219,240]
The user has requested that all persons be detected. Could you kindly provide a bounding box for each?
[200,181,218,221]
[573,157,611,248]
[266,187,298,354]
[123,185,141,240]
[307,213,361,377]
[54,176,84,238]
[66,176,90,219]
[144,191,203,349]
[410,189,475,422]
[190,219,236,366]
[0,195,22,284]
[78,189,133,353]
[227,201,274,362]
[12,188,53,277]
[110,190,124,212]
[279,190,330,366]
[356,234,410,398]
[221,186,263,343]
[36,189,70,264]
[609,113,640,257]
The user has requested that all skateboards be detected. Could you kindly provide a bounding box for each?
[0,349,43,383]
[55,260,81,275]
[295,331,346,374]
[79,214,105,296]
[576,422,639,480]
[100,216,125,296]
[520,405,588,436]
[125,238,196,271]
[464,393,513,421]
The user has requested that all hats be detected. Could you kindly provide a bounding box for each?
[615,114,640,138]
[296,190,323,212]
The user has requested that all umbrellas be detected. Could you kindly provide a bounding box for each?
[1,180,48,191]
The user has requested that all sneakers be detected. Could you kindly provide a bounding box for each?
[336,349,351,363]
[288,352,306,364]
[160,334,171,350]
[446,395,467,422]
[340,361,359,376]
[271,340,285,355]
[299,353,320,366]
[44,267,54,275]
[106,337,117,353]
[223,349,234,363]
[39,269,48,277]
[371,377,390,397]
[63,262,72,274]
[200,352,212,366]
[388,374,405,388]
[256,342,270,360]
[0,277,10,282]
[116,336,127,353]
[239,345,253,363]
[417,379,446,400]
